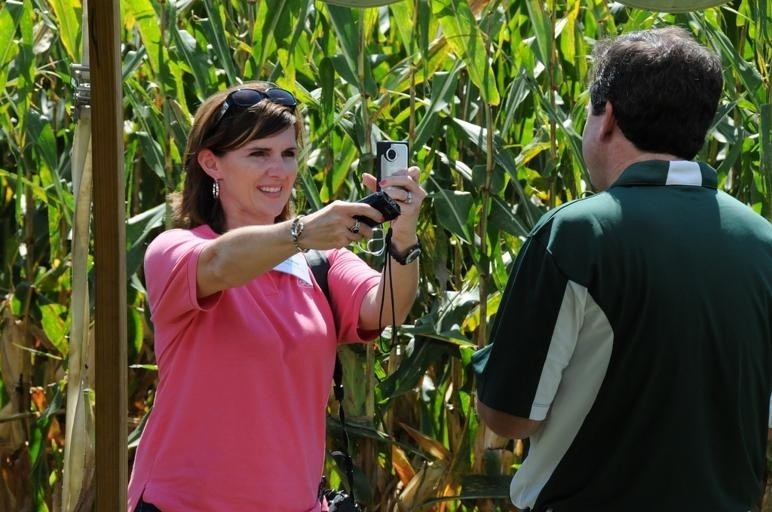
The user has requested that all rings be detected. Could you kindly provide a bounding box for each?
[407,192,412,204]
[351,222,360,233]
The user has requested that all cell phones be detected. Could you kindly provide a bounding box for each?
[375,141,411,201]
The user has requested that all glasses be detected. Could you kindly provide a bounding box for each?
[206,87,297,133]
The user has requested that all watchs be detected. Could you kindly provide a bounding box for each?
[385,228,420,266]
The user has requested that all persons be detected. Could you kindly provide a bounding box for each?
[479,28,772,512]
[126,81,420,512]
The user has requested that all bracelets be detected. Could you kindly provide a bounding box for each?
[288,215,309,254]
